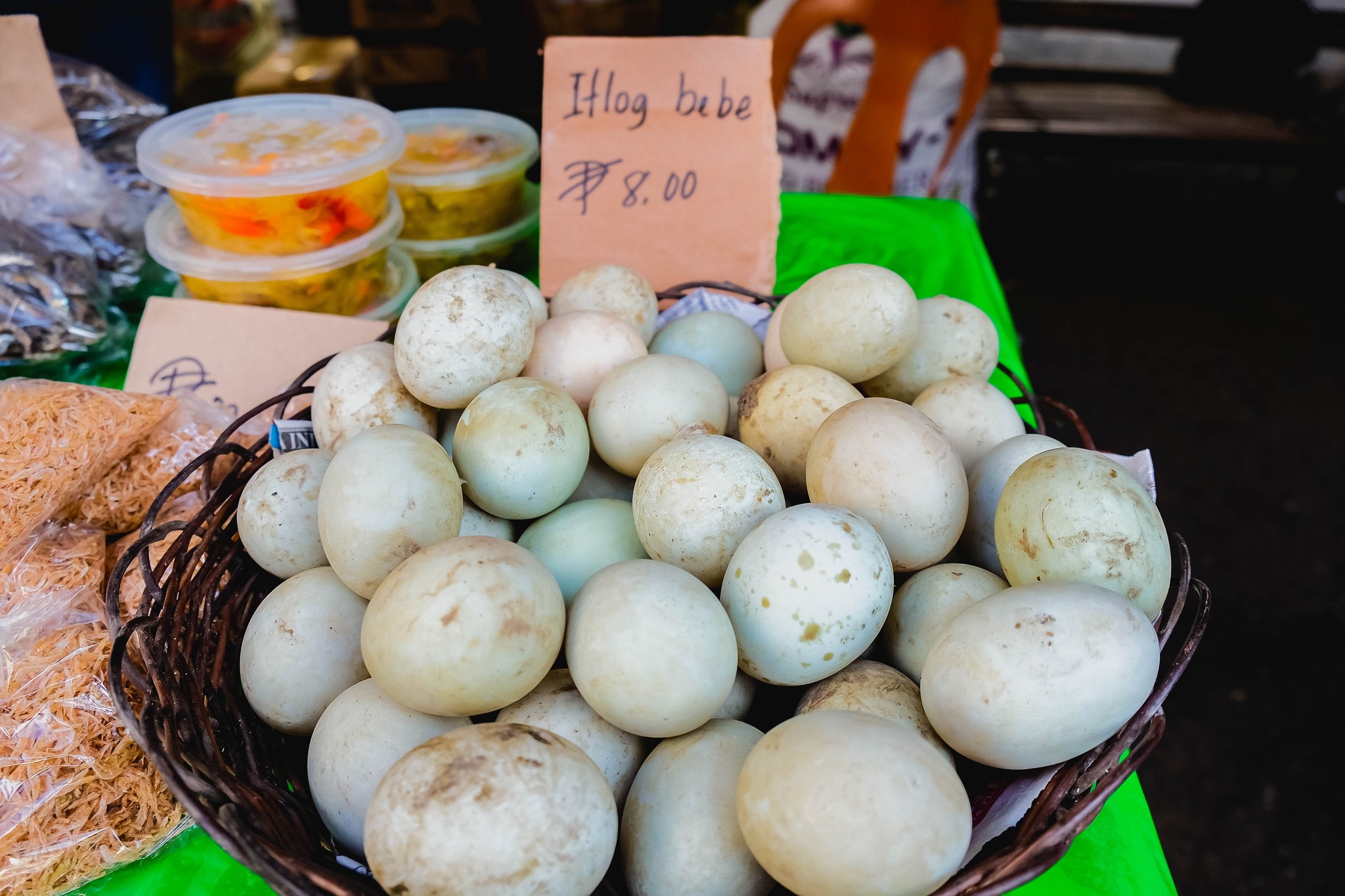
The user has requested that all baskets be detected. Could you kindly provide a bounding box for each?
[100,270,1202,896]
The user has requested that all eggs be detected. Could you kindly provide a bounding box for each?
[235,263,1172,896]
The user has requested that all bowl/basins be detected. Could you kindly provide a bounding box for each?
[134,91,541,340]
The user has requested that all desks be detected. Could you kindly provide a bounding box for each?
[66,192,1178,896]
